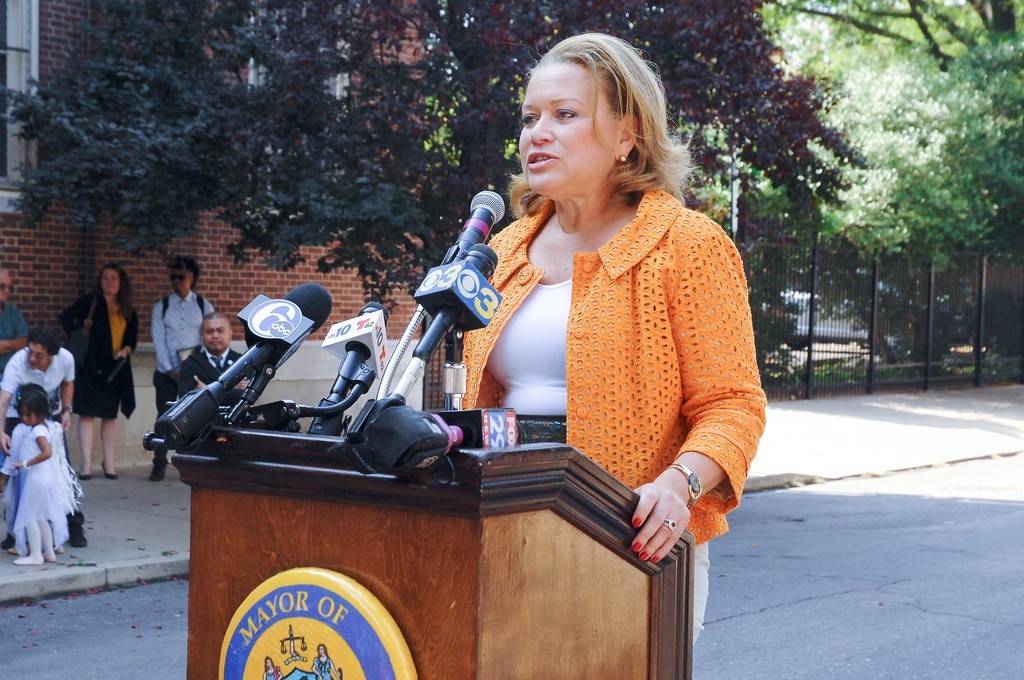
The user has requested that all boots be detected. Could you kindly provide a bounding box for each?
[0,532,16,550]
[66,511,88,548]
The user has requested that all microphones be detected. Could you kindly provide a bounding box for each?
[345,242,499,444]
[155,282,333,449]
[308,301,390,437]
[415,190,506,316]
[436,408,525,451]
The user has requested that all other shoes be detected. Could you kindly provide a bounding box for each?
[101,461,117,480]
[78,473,92,480]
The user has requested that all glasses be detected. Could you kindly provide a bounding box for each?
[170,273,192,281]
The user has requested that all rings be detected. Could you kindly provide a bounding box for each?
[663,519,677,533]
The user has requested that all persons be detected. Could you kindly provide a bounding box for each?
[0,264,138,565]
[149,256,250,481]
[461,31,768,649]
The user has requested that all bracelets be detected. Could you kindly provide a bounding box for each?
[22,459,30,470]
[61,406,73,415]
[122,348,128,354]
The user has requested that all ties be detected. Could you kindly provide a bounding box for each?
[210,357,222,370]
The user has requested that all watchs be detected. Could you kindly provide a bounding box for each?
[670,462,703,509]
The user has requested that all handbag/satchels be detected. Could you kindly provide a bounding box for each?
[65,326,90,355]
